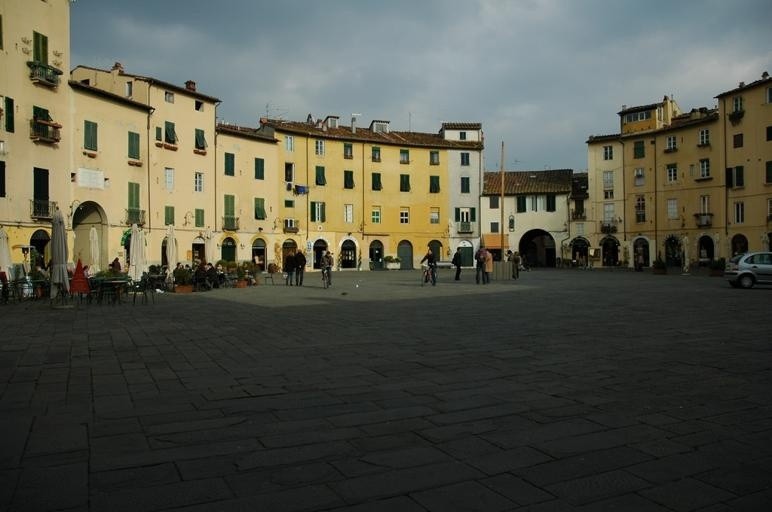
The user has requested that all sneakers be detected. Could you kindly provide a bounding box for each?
[296,282,302,286]
[512,278,519,281]
[322,278,331,285]
[476,280,489,285]
[455,278,460,281]
[286,283,293,286]
[425,280,435,286]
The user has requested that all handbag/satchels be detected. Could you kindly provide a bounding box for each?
[280,272,289,280]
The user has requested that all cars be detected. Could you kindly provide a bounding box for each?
[724,251,772,289]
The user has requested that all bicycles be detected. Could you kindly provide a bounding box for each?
[422,264,439,286]
[323,265,330,289]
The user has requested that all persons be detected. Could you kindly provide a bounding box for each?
[633,253,645,272]
[506,249,532,281]
[294,247,306,287]
[319,250,334,287]
[451,246,464,280]
[484,248,494,284]
[282,250,295,287]
[473,245,487,285]
[0,252,259,306]
[419,248,437,287]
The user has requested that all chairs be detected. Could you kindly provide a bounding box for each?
[5,276,156,307]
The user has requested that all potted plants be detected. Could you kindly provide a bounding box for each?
[173,268,194,294]
[234,266,248,288]
[384,254,401,271]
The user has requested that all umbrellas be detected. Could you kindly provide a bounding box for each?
[202,225,215,265]
[139,229,152,274]
[46,207,72,304]
[125,221,144,282]
[0,228,13,281]
[162,224,178,283]
[84,224,101,278]
